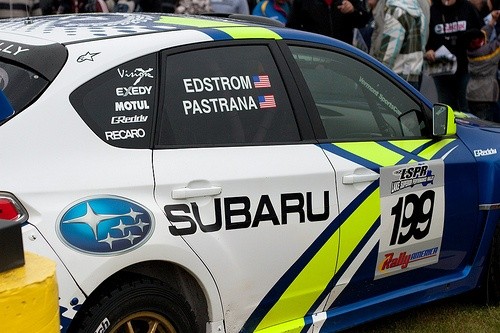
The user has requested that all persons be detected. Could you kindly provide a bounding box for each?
[252,0,289,26]
[209,0,250,15]
[370,0,423,94]
[286,0,369,44]
[425,0,500,118]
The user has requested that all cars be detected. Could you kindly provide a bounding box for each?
[0,12,500,333]
